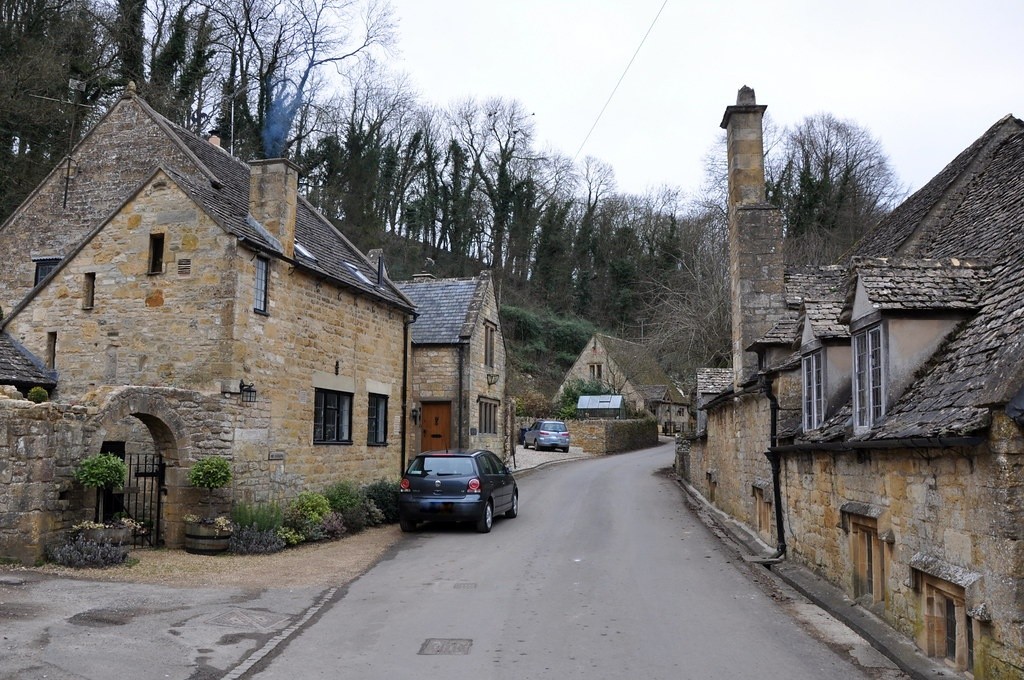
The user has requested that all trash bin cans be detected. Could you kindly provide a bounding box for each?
[520,427,533,445]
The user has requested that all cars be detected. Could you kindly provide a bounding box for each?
[396,447,520,533]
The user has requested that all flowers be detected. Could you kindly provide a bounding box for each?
[72,517,146,538]
[182,514,233,536]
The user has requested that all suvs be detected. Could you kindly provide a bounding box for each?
[523,419,571,453]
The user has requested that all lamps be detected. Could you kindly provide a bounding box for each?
[221,379,256,402]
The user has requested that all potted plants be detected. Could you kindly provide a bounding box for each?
[182,456,233,556]
[72,451,146,561]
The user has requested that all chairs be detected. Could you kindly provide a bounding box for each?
[552,426,557,429]
[545,427,548,430]
